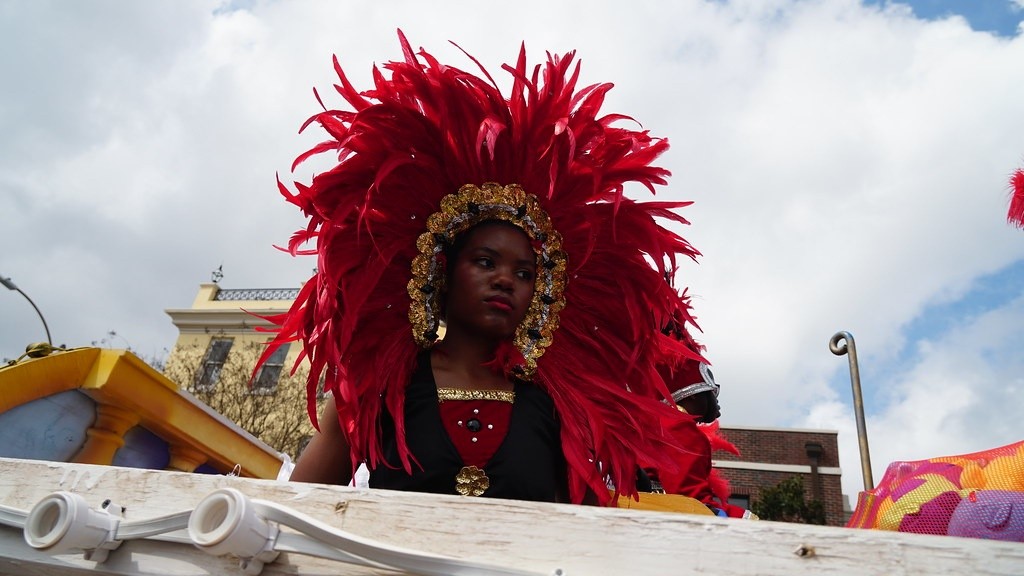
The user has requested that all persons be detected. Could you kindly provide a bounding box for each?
[637,387,759,521]
[290,181,600,503]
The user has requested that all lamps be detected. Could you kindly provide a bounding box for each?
[205,262,228,285]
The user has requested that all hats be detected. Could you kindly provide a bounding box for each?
[627,267,719,406]
